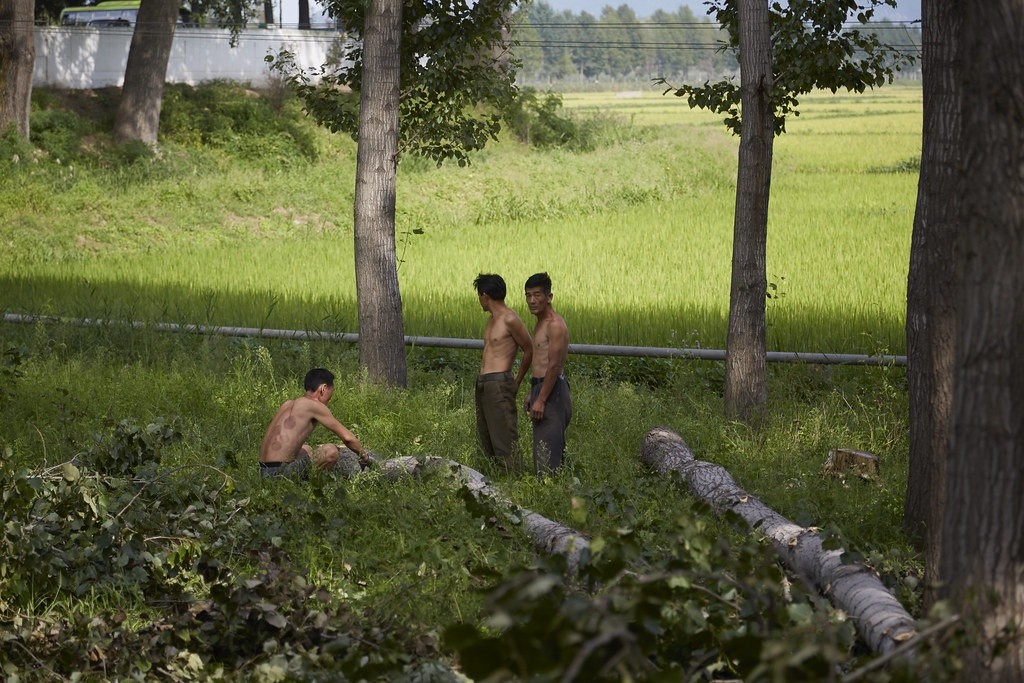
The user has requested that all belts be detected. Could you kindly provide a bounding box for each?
[477,372,513,381]
[259,462,284,467]
[532,375,563,385]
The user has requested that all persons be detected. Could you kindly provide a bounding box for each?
[257,367,377,490]
[522,271,574,478]
[469,271,534,475]
